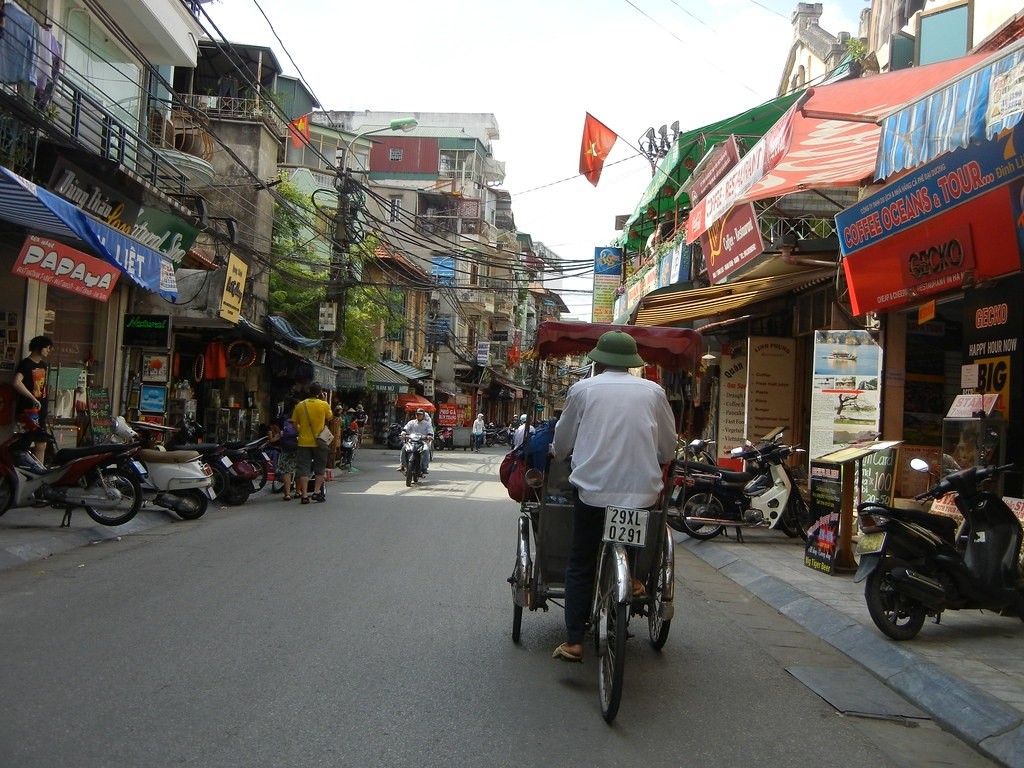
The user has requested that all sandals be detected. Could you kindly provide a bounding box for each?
[301,496,309,503]
[294,492,302,498]
[312,493,326,501]
[283,494,291,500]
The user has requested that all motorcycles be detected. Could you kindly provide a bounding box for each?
[0,399,148,526]
[663,428,812,544]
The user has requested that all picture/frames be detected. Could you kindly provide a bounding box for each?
[139,385,166,413]
[141,354,169,382]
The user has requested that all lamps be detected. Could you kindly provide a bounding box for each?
[165,191,208,225]
[194,215,242,244]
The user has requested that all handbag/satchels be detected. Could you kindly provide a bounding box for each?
[500,452,532,500]
[317,425,334,447]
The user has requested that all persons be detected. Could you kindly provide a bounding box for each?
[13,335,52,466]
[508,414,520,447]
[396,408,434,474]
[290,382,333,504]
[550,329,679,659]
[333,401,368,472]
[513,414,536,449]
[260,398,303,501]
[472,413,485,453]
[656,210,679,243]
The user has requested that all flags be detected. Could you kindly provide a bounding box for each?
[578,113,616,186]
[288,114,310,148]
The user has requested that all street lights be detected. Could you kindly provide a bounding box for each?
[321,117,419,365]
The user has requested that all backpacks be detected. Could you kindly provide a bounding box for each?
[280,418,299,451]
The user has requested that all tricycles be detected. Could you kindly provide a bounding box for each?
[506,416,677,725]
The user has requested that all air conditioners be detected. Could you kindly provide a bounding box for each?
[153,115,174,149]
[402,347,414,362]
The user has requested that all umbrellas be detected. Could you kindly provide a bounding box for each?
[394,394,436,410]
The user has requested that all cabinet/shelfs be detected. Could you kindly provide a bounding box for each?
[204,407,260,443]
[943,417,1007,500]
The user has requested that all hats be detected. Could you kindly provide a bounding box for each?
[588,330,644,368]
[347,408,356,412]
[336,405,343,410]
[416,409,425,415]
[520,414,526,421]
[357,404,364,410]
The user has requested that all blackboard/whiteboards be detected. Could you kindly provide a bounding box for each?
[852,448,900,510]
[87,387,113,445]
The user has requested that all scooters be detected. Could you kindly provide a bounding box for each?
[384,423,516,450]
[98,414,216,521]
[854,456,1024,641]
[397,433,432,487]
[139,427,275,506]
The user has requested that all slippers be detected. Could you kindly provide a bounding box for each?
[633,581,645,596]
[552,643,583,662]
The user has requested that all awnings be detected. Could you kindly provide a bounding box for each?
[684,54,996,249]
[0,165,179,303]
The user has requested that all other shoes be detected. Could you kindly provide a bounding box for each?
[396,466,404,471]
[422,468,428,474]
